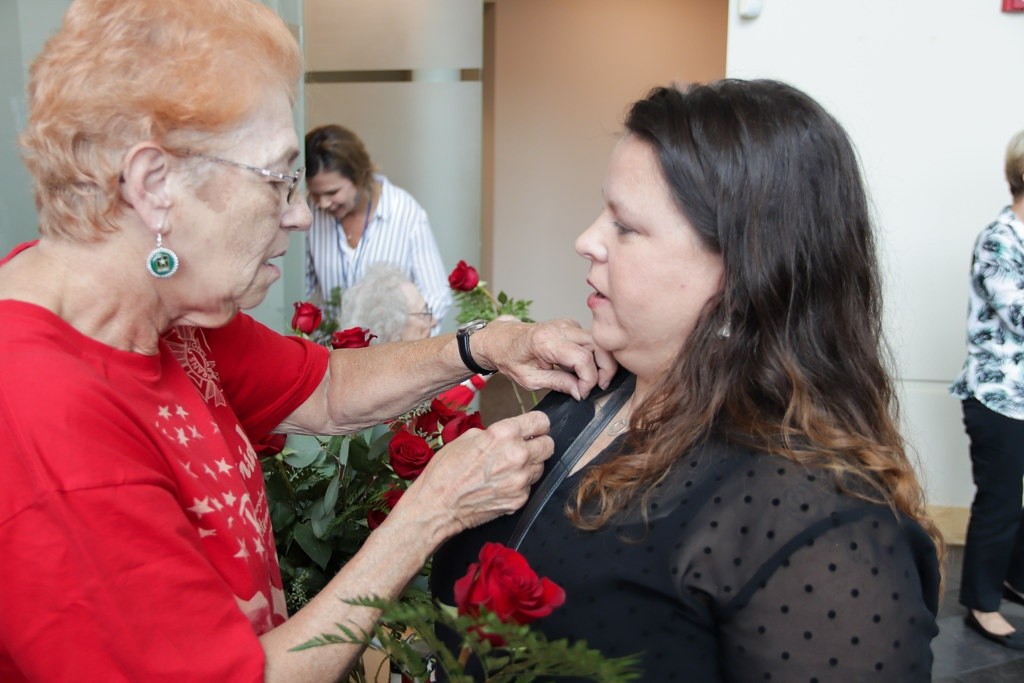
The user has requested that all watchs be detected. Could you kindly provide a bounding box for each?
[456,319,498,375]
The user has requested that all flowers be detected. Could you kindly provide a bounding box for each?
[255,260,645,683]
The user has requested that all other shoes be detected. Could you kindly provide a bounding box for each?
[969,610,1024,648]
[1002,580,1024,606]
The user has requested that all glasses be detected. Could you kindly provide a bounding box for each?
[119,150,306,205]
[406,303,433,321]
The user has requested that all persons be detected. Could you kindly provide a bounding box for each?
[429,76,950,683]
[0,0,618,683]
[301,124,452,350]
[947,128,1024,651]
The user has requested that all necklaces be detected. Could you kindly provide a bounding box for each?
[607,394,634,436]
